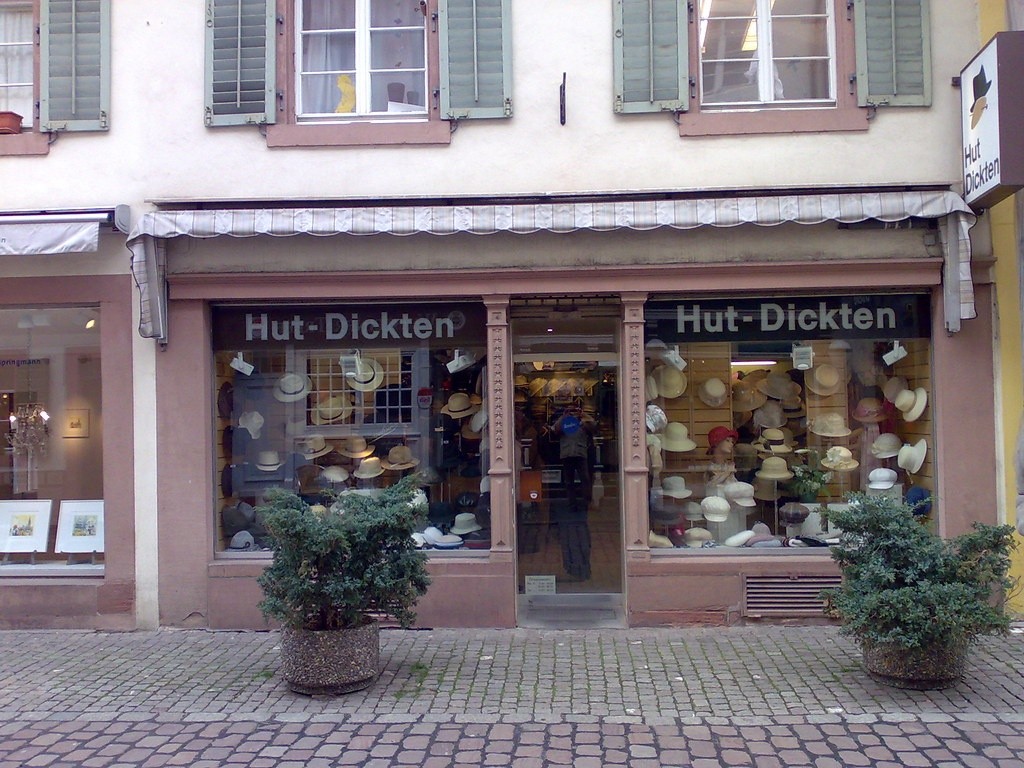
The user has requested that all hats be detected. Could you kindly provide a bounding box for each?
[219,354,607,551]
[561,415,580,435]
[642,361,933,546]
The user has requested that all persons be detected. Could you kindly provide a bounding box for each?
[531,396,597,582]
[706,425,739,464]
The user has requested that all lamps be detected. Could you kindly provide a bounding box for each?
[660,344,687,370]
[229,350,255,377]
[791,340,815,369]
[883,337,908,366]
[740,0,776,51]
[446,346,474,374]
[339,346,361,378]
[4,313,50,456]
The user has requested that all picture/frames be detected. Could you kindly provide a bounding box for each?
[55,500,105,553]
[61,408,90,439]
[0,499,52,553]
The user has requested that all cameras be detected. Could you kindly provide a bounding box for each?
[568,409,578,413]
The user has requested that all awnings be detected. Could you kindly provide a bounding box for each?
[126,191,976,345]
[0,214,111,256]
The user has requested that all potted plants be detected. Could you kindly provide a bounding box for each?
[256,469,434,695]
[812,489,1024,693]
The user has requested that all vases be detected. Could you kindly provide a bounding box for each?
[0,111,24,135]
